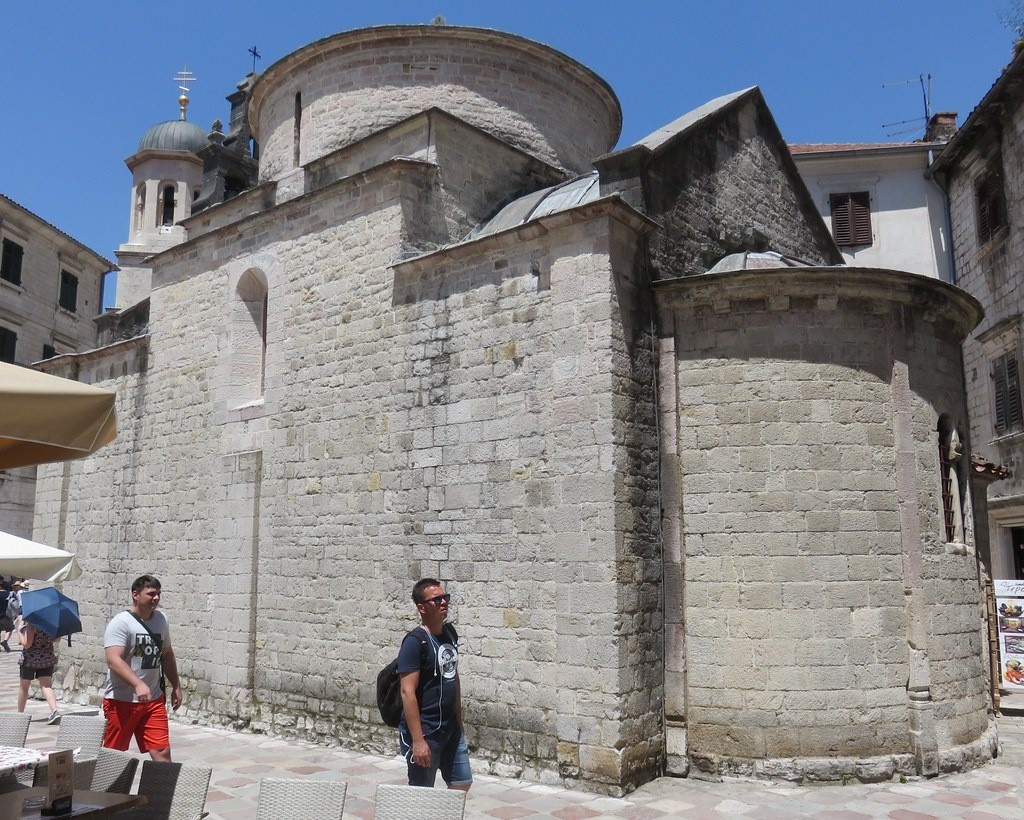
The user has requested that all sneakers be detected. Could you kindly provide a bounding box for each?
[1,641,10,653]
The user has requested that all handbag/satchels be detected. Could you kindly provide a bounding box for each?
[160,675,167,703]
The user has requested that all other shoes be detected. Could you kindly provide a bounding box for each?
[47,710,62,725]
[201,812,209,819]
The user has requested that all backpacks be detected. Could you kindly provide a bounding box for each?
[377,624,456,729]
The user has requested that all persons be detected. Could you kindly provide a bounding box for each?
[400,578,473,794]
[0,576,30,653]
[99,575,182,762]
[17,616,62,725]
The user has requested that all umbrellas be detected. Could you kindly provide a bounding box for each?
[20,586,83,640]
[0,530,83,583]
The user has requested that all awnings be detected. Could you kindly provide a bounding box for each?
[0,361,118,471]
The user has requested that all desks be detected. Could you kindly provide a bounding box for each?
[0,784,149,820]
[0,743,60,779]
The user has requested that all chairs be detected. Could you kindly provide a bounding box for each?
[110,760,213,820]
[374,783,467,820]
[54,715,110,792]
[255,777,347,820]
[0,711,32,748]
[88,746,140,795]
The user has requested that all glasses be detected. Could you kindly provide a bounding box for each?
[421,594,451,605]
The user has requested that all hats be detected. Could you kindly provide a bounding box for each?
[11,581,21,586]
[21,580,30,588]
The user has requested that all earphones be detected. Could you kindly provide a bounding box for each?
[453,641,457,647]
[434,669,437,676]
